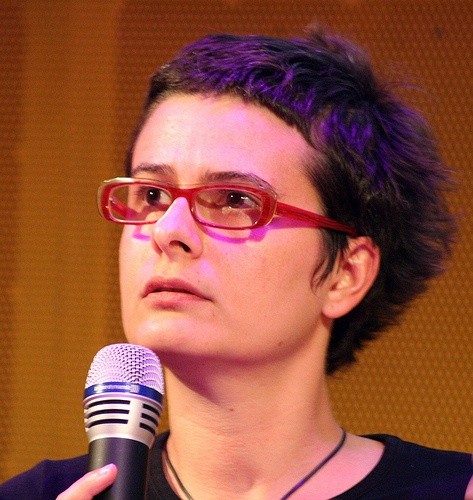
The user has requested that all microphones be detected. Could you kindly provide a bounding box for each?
[83,343,165,500]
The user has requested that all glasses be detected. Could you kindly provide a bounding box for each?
[97,177,359,240]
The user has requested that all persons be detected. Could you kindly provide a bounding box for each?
[0,17,472,499]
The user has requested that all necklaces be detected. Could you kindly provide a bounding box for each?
[161,424,346,500]
